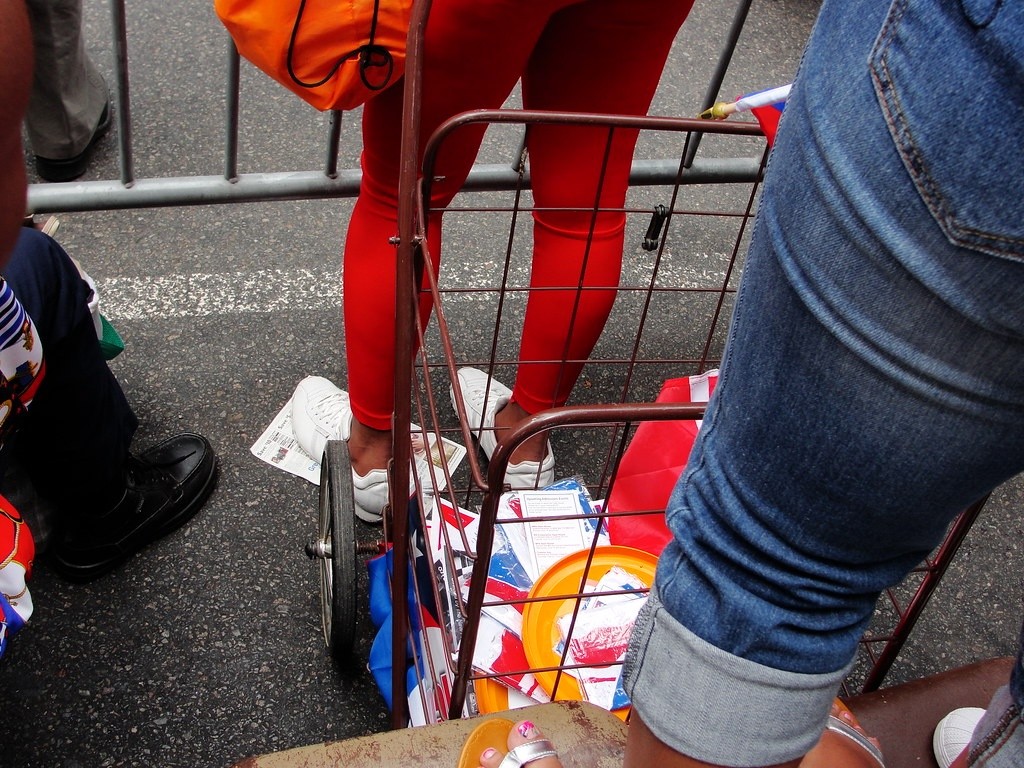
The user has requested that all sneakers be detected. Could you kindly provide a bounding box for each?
[292,375,421,524]
[450,366,555,488]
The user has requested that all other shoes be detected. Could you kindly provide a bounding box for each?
[36,73,111,183]
[933,707,987,768]
[51,433,216,582]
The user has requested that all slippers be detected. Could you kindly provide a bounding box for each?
[798,696,886,768]
[457,718,564,768]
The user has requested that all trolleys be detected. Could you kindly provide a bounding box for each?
[305,1,991,730]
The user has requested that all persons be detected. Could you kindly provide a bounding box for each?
[291,0,696,524]
[460,0,1024,768]
[0,0,221,587]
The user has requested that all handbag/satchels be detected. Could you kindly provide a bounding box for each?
[215,0,414,110]
